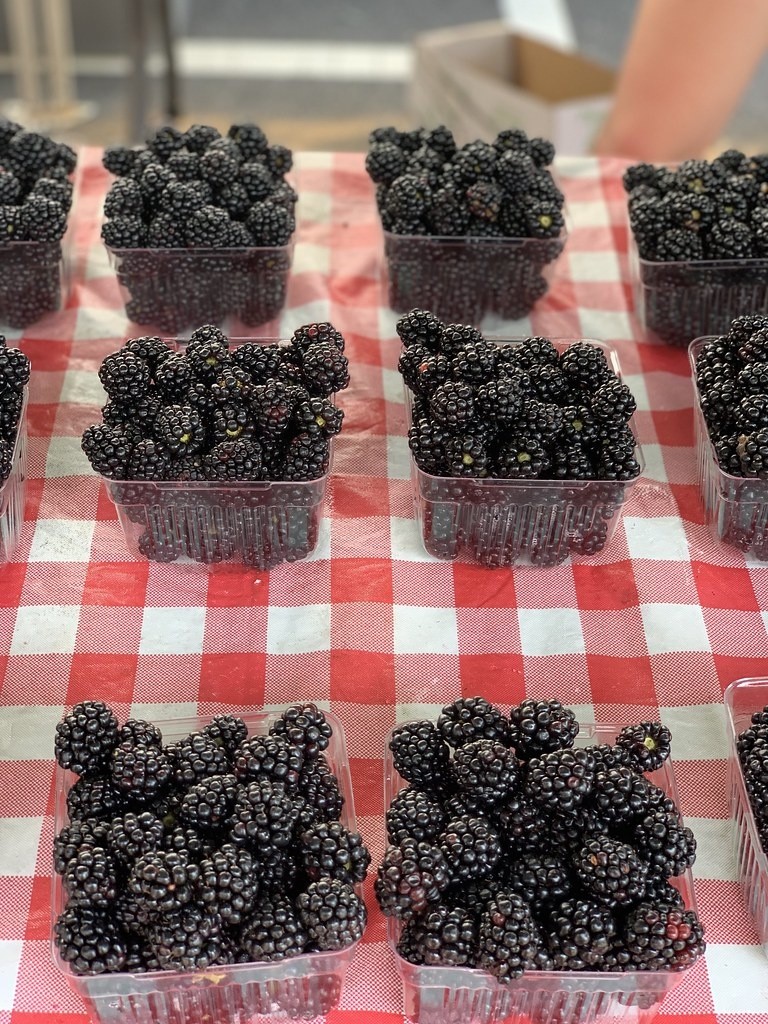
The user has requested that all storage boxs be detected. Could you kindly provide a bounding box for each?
[686,334,768,560]
[87,331,353,574]
[41,698,365,1024]
[719,670,768,953]
[0,131,88,328]
[395,326,650,570]
[96,143,304,331]
[630,176,768,355]
[0,333,44,562]
[405,19,617,150]
[380,708,709,1024]
[373,153,578,323]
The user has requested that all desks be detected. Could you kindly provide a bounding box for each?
[0,150,768,1024]
